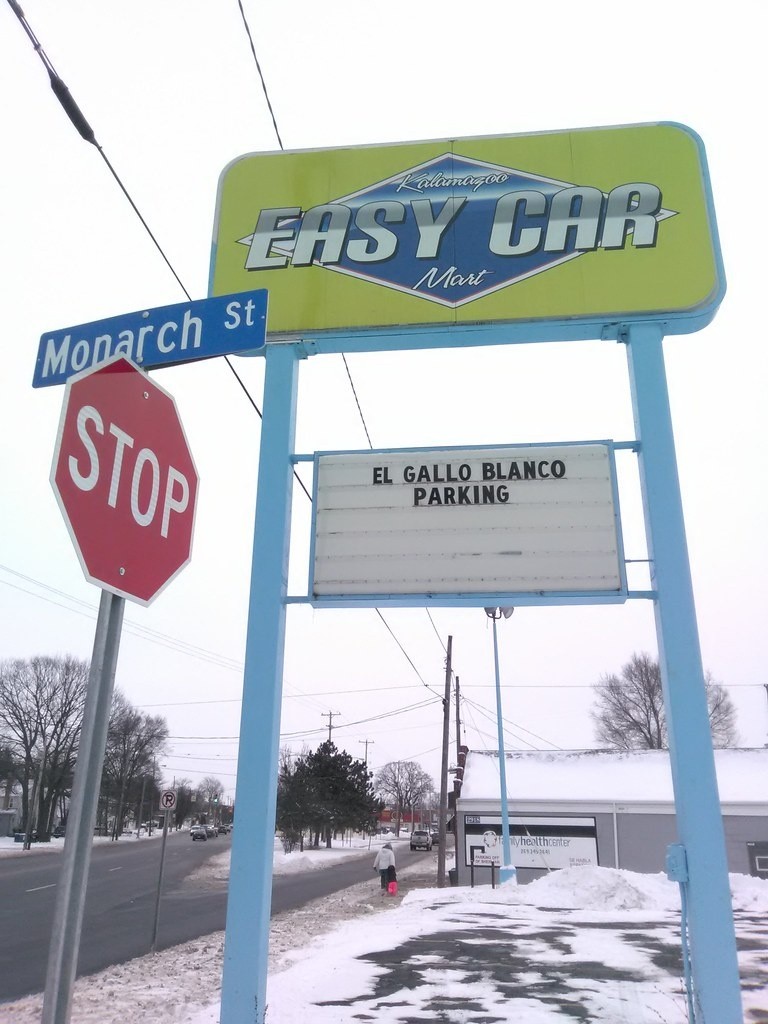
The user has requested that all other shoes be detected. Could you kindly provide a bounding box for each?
[381,891,389,897]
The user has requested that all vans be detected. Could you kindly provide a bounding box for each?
[432,833,440,845]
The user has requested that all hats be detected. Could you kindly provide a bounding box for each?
[385,842,391,848]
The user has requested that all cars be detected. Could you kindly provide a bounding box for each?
[190,822,233,841]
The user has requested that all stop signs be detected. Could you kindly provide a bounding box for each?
[47,351,201,608]
[158,789,178,810]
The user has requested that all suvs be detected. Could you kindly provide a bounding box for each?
[410,830,433,850]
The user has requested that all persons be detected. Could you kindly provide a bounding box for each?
[372,843,396,890]
